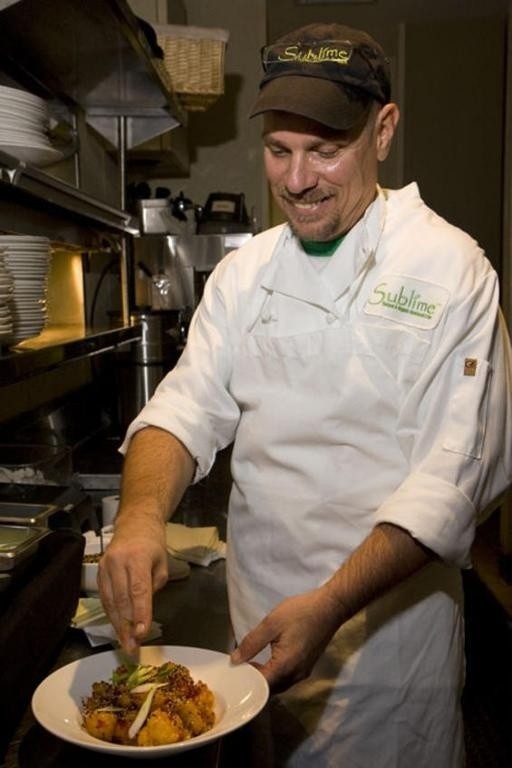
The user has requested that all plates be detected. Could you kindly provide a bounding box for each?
[0,236,55,348]
[0,85,64,167]
[31,645,269,758]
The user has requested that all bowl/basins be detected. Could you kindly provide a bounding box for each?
[81,543,108,598]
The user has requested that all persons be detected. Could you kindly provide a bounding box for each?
[92,19,511,767]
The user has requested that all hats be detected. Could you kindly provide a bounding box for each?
[248,21,392,131]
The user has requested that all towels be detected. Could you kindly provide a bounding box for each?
[164,521,226,567]
